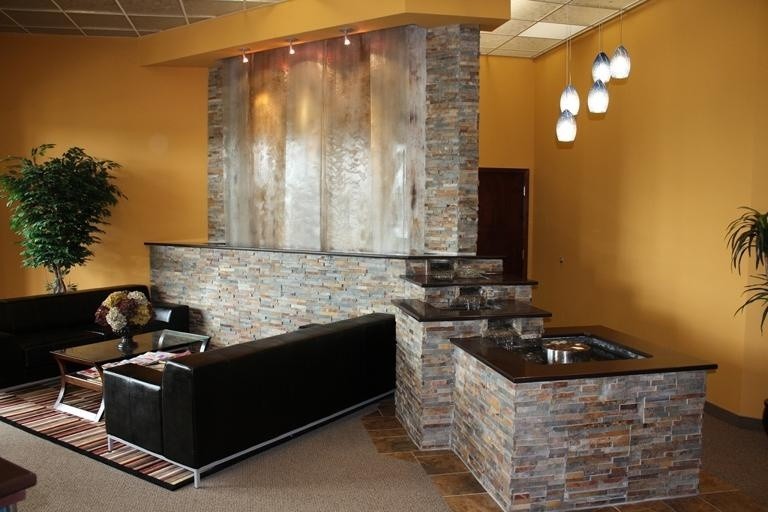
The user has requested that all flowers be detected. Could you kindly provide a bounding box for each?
[95,290,157,331]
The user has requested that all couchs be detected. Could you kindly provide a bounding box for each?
[102,313,395,488]
[0,284,189,390]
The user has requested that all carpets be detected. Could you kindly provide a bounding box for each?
[0,372,394,491]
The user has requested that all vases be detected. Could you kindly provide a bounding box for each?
[112,324,140,348]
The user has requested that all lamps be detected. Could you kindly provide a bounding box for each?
[555,0,631,142]
